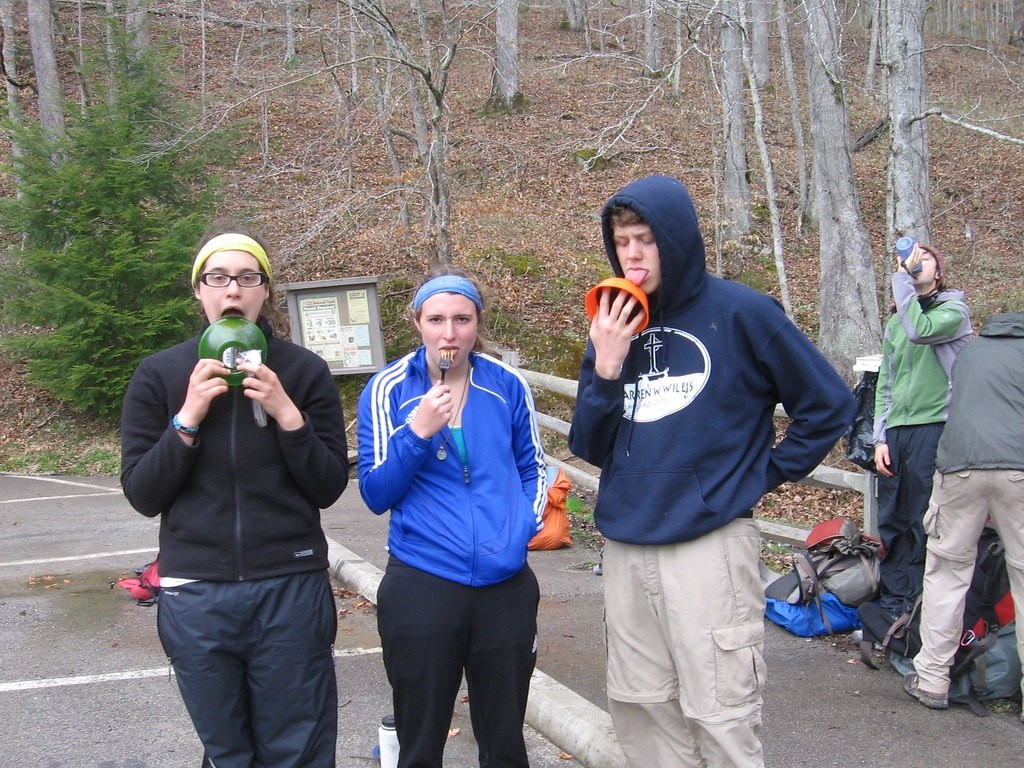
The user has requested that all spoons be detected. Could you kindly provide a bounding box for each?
[245,350,266,427]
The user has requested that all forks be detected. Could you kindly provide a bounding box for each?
[439,350,450,399]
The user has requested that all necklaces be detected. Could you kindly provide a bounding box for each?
[437,363,470,461]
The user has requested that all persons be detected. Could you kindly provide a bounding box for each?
[871,243,972,619]
[568,175,859,768]
[357,269,549,768]
[901,310,1024,723]
[120,223,350,768]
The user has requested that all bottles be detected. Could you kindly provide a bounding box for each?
[965,227,971,242]
[379,715,400,768]
[896,237,923,273]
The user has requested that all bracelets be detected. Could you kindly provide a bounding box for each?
[172,414,199,438]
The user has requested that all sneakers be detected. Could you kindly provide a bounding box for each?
[902,671,948,709]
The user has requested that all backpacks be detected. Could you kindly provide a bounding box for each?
[805,516,882,608]
[858,525,1022,704]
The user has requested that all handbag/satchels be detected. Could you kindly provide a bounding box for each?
[841,370,881,475]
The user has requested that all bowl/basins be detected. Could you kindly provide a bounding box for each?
[198,317,268,386]
[585,278,649,335]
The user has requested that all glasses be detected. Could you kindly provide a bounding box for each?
[199,272,269,288]
